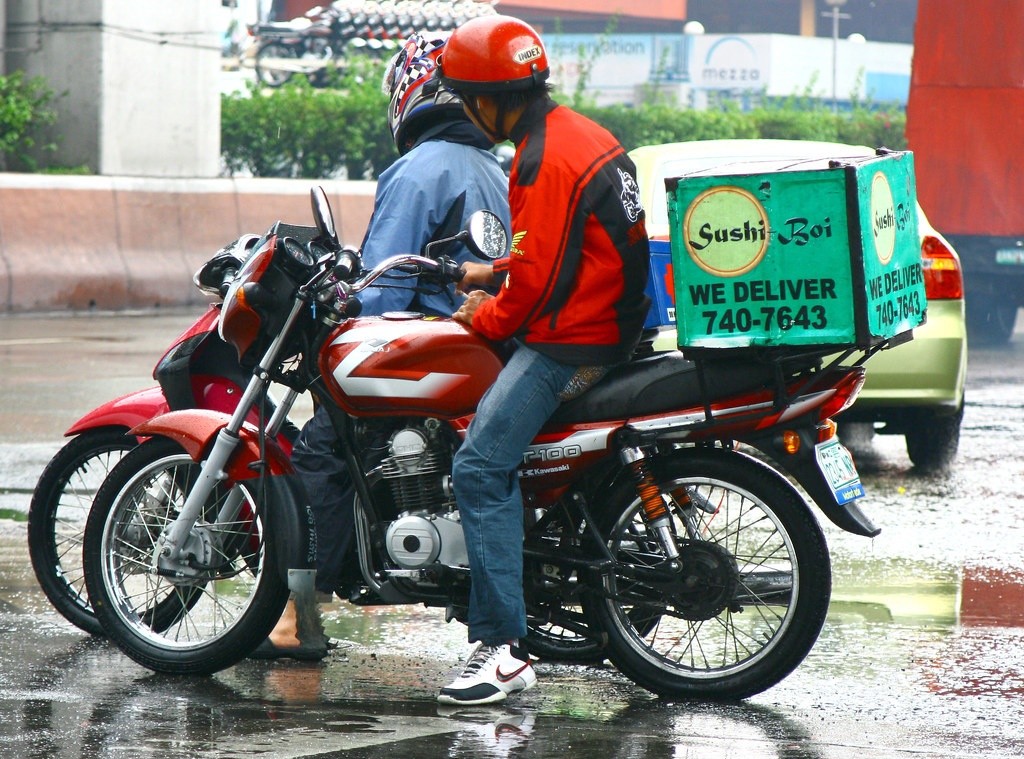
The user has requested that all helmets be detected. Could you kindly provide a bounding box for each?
[381,30,464,157]
[436,14,551,92]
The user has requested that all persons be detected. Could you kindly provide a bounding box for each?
[249,32,512,661]
[435,14,651,707]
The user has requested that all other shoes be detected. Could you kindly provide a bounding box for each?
[243,634,329,661]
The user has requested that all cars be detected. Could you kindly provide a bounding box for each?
[626,139,967,472]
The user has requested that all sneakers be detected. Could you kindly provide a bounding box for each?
[436,641,538,705]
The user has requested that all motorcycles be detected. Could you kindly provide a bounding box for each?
[26,229,669,664]
[83,186,913,701]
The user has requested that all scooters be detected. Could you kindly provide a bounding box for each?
[245,0,501,88]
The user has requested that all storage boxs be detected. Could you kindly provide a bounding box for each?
[665,145,928,351]
